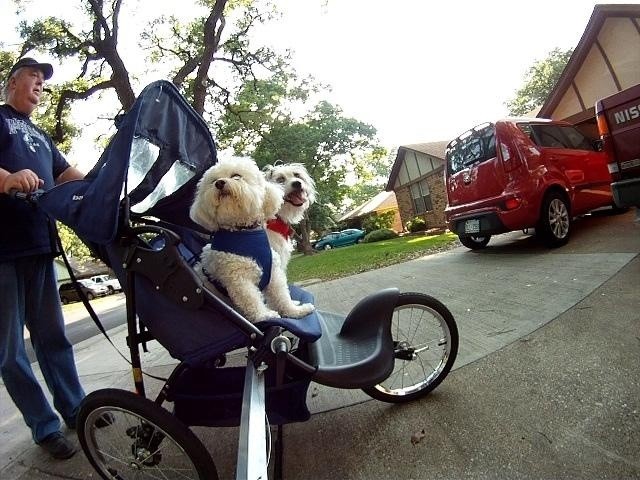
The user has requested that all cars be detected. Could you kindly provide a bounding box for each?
[314,228,367,251]
[56,272,123,304]
[442,118,628,248]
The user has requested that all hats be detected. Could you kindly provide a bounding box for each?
[9,58,57,80]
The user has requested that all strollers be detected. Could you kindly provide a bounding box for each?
[13,82,460,479]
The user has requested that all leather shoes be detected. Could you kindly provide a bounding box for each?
[39,431,76,459]
[67,410,113,430]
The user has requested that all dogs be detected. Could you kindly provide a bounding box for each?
[188,154,321,323]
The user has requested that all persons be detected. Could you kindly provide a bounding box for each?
[0,57,114,463]
